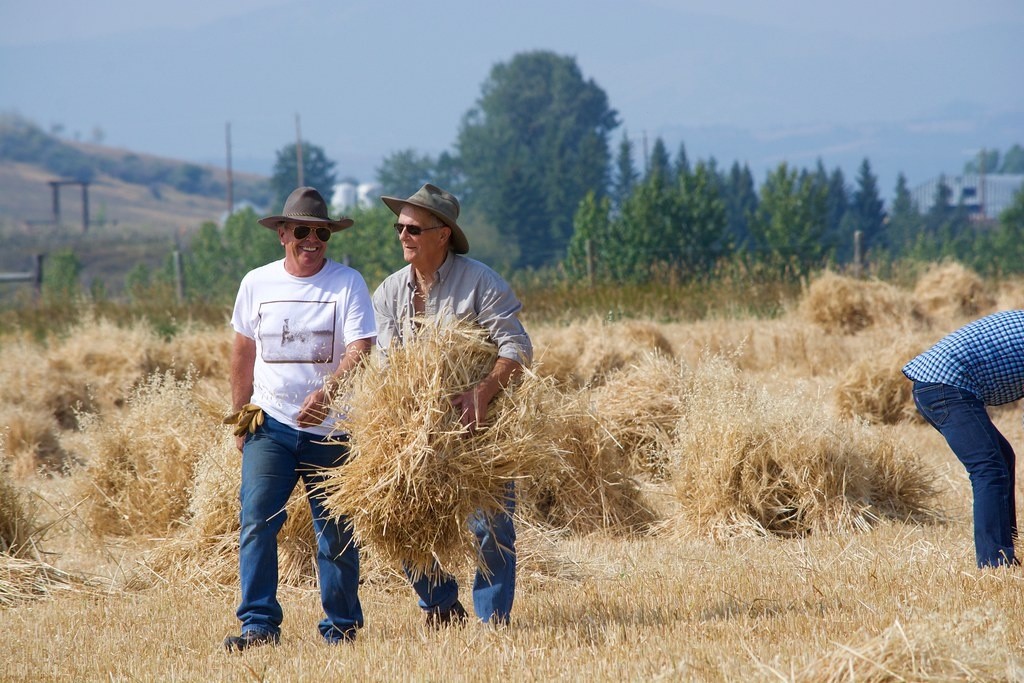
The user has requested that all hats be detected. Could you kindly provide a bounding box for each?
[381,183,469,254]
[257,186,354,234]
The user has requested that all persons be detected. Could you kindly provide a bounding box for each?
[903,308,1024,568]
[223,186,378,654]
[372,182,533,627]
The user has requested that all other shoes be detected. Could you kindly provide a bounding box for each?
[425,605,469,632]
[224,630,278,654]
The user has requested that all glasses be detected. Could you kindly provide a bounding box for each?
[394,223,449,235]
[284,225,331,242]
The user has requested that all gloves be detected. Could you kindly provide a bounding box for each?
[224,404,264,438]
